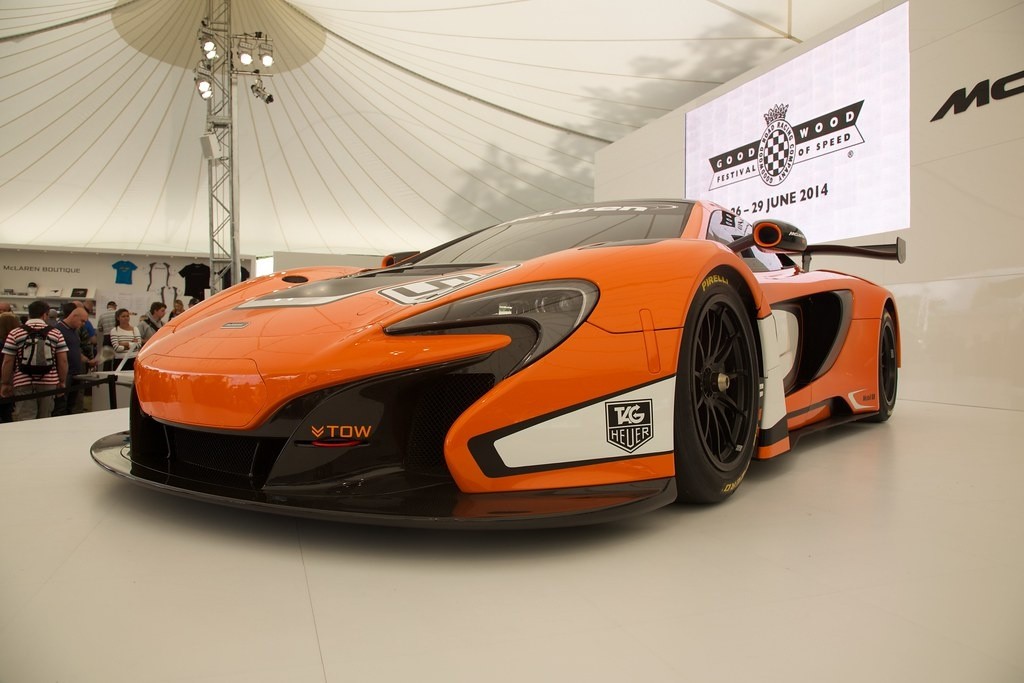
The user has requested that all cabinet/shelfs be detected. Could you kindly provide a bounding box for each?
[0,294,98,316]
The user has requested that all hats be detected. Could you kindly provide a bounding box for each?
[59,302,77,320]
[108,301,117,306]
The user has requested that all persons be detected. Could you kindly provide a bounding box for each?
[110,308,141,371]
[168,300,185,323]
[63,303,95,374]
[188,298,199,308]
[0,300,70,422]
[53,307,96,417]
[97,301,118,371]
[84,299,94,312]
[47,309,60,326]
[137,301,167,343]
[0,302,20,341]
[72,300,97,344]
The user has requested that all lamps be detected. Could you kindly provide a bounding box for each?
[251,74,274,104]
[194,74,213,100]
[236,39,254,65]
[257,39,274,67]
[198,28,216,59]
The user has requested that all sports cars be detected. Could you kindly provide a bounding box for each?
[89,201,903,532]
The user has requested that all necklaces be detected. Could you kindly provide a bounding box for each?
[61,320,71,329]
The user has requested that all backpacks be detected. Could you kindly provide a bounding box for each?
[18,324,56,381]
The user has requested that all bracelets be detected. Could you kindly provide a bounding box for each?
[86,359,89,365]
